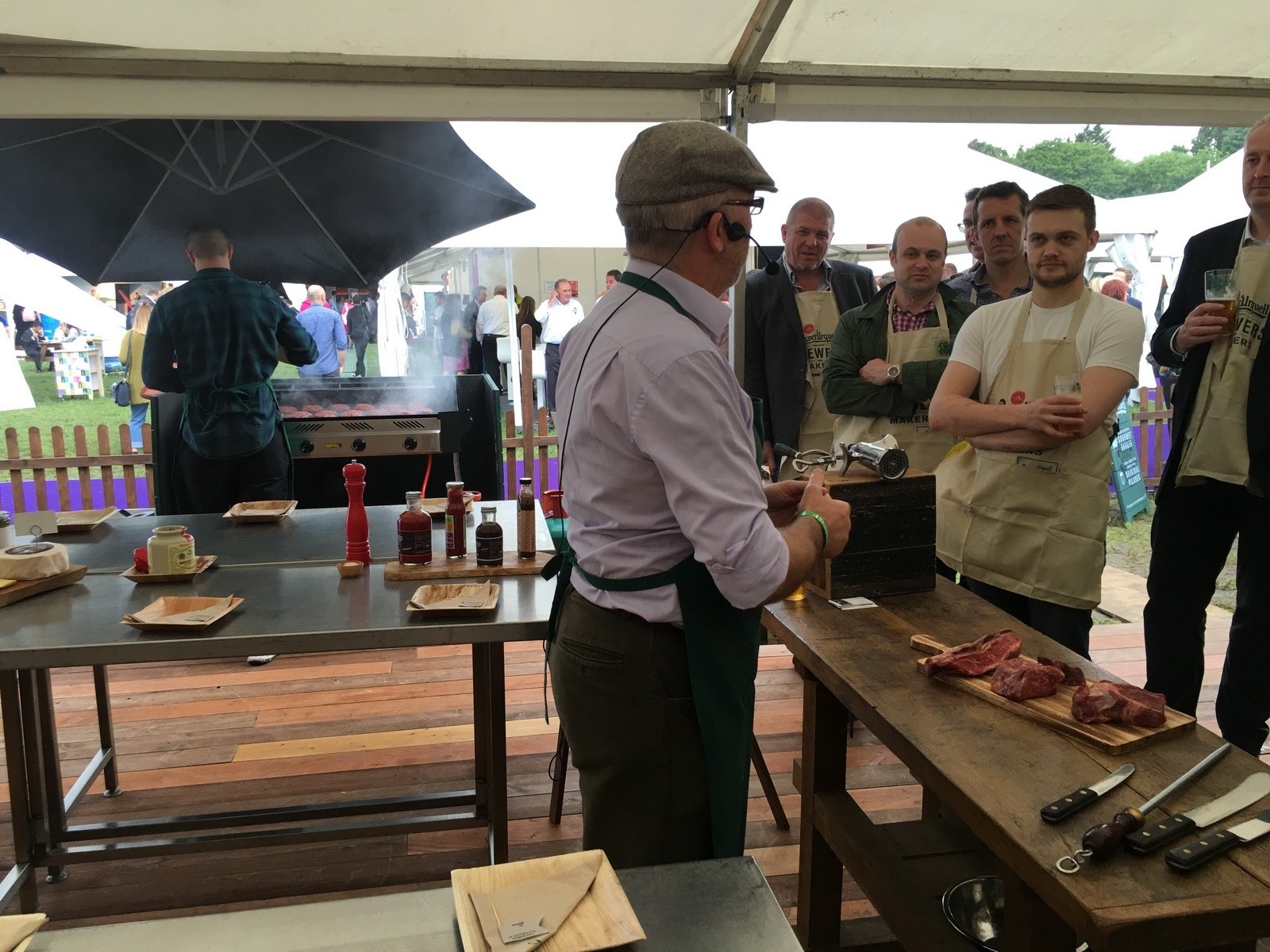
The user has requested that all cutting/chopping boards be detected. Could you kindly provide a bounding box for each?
[910,634,1197,755]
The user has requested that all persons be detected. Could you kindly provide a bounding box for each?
[1089,268,1143,309]
[142,224,319,514]
[91,290,99,299]
[746,197,878,484]
[820,216,976,470]
[942,180,1033,307]
[119,286,173,454]
[548,119,851,871]
[534,278,584,427]
[596,270,621,305]
[14,305,79,373]
[279,283,541,396]
[927,184,1146,661]
[1145,115,1269,757]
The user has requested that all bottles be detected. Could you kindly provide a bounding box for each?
[397,491,432,566]
[445,481,467,558]
[343,459,371,566]
[475,505,503,567]
[517,477,537,560]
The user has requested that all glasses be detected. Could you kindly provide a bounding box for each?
[723,196,764,217]
[958,222,974,233]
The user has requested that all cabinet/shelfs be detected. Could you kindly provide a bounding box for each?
[51,348,105,400]
[759,574,1270,952]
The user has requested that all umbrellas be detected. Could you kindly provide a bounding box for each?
[1,118,535,290]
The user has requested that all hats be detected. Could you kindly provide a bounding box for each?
[616,120,777,205]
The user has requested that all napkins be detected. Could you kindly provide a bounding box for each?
[424,496,474,513]
[468,854,604,952]
[0,912,50,952]
[405,578,490,610]
[229,501,296,515]
[128,592,234,623]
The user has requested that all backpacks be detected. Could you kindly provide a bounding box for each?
[22,308,36,322]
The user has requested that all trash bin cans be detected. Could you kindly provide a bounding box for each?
[40,313,60,340]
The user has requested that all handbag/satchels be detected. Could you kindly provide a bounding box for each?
[367,329,376,343]
[109,379,130,407]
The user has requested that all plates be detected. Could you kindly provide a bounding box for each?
[54,509,118,531]
[222,500,297,524]
[118,596,245,630]
[450,849,646,952]
[420,498,473,517]
[119,554,218,584]
[0,913,47,952]
[406,583,500,615]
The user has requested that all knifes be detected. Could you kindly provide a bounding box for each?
[1126,771,1270,870]
[1040,763,1135,823]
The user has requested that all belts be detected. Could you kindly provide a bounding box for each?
[484,333,506,337]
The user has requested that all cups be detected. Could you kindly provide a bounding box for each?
[1204,268,1239,337]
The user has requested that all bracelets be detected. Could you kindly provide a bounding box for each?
[800,511,827,551]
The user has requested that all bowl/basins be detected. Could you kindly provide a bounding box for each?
[941,873,1088,952]
[336,561,364,577]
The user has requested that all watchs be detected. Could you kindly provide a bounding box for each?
[887,364,901,384]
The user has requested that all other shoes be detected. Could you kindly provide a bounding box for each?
[48,367,55,371]
[37,369,42,373]
[246,656,277,663]
[351,373,362,377]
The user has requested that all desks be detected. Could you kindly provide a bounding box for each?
[38,341,63,362]
[25,856,803,952]
[0,494,563,913]
